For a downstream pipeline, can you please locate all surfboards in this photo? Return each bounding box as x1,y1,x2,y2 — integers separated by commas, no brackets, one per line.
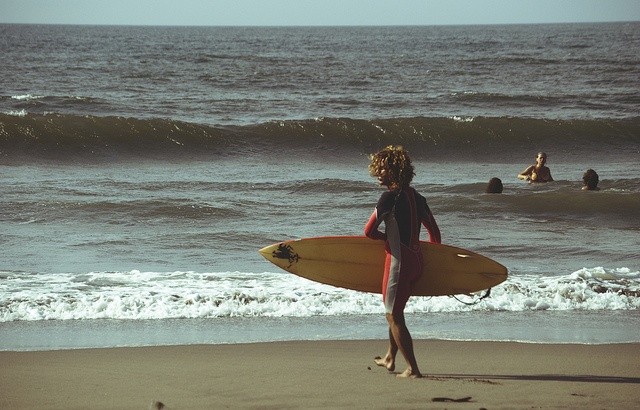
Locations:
258,235,509,297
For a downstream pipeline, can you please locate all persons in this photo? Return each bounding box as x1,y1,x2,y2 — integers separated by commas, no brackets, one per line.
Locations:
518,153,555,184
365,145,442,379
581,168,602,191
485,176,503,193
